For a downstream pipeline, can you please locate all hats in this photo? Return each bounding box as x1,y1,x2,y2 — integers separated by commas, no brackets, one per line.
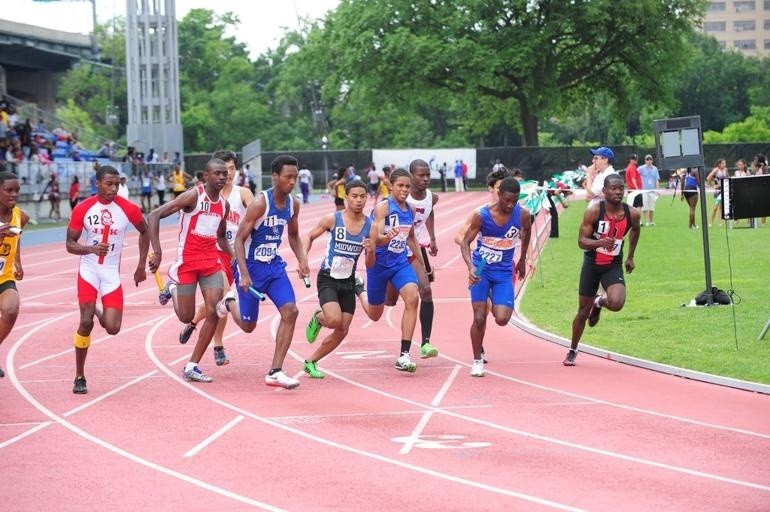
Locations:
589,147,615,162
628,153,638,160
644,155,653,160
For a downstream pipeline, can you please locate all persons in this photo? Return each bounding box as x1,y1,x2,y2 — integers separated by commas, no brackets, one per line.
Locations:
237,164,257,197
295,162,398,204
561,173,642,367
196,170,207,185
667,176,681,190
146,157,236,384
459,174,534,378
439,160,470,192
453,166,538,368
354,166,428,373
301,178,379,379
215,153,311,389
66,163,151,395
0,168,31,378
177,148,257,368
680,153,769,229
492,147,617,209
402,158,439,360
2,91,194,222
626,153,660,227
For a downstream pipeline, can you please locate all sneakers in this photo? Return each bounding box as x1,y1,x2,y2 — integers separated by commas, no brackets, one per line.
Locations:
73,376,88,394
395,343,439,373
303,307,327,380
481,352,488,363
158,274,239,384
563,350,578,366
587,295,602,328
470,359,484,377
264,369,300,390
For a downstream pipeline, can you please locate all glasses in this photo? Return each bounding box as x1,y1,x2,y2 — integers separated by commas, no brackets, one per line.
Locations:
647,158,653,160
